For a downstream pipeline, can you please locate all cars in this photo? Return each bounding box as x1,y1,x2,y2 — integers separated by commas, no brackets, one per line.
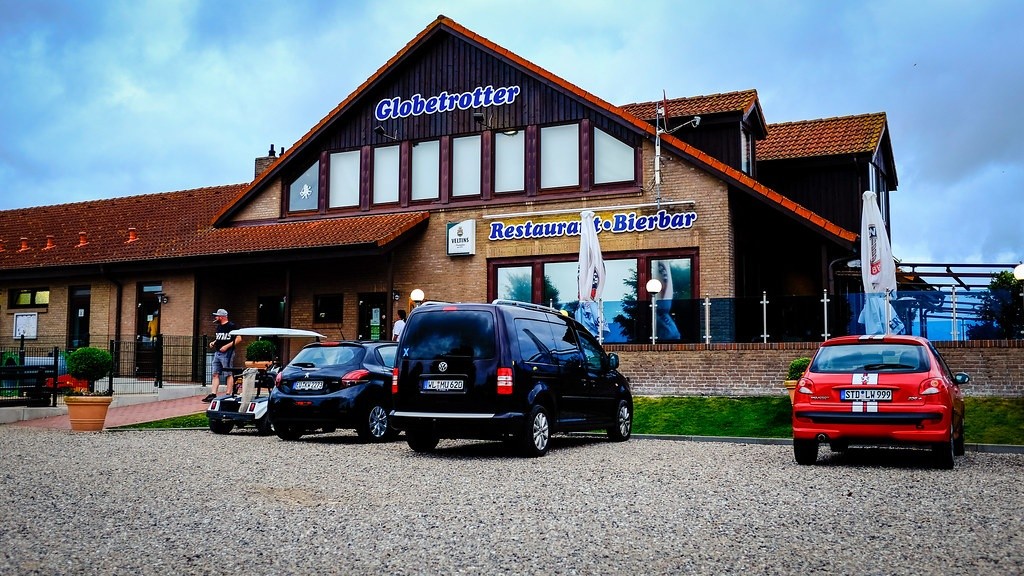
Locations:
266,340,400,443
793,335,970,469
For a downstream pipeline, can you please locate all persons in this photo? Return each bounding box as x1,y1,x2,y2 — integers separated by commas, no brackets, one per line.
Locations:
202,309,242,402
392,310,406,342
148,309,158,342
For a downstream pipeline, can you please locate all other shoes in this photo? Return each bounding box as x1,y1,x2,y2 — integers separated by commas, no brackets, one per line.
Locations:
202,394,216,403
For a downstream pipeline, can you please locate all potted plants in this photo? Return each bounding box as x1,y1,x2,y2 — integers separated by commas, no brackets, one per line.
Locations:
244,340,277,370
784,357,813,407
64,348,113,432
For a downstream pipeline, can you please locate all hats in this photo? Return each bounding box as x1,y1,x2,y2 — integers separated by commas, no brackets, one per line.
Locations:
212,309,229,316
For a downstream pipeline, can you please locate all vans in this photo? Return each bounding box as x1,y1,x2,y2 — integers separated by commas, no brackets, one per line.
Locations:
388,298,634,456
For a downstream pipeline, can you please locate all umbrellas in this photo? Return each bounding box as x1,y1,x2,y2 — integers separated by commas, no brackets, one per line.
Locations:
575,211,610,335
858,191,904,335
651,260,679,339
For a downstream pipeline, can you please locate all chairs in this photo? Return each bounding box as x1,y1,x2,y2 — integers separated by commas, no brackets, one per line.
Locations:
900,350,919,369
862,353,886,367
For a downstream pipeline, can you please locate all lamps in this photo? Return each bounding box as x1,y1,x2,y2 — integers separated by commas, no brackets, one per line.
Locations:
646,279,662,297
410,289,425,305
154,292,168,304
374,125,398,141
1014,264,1024,286
474,113,492,129
